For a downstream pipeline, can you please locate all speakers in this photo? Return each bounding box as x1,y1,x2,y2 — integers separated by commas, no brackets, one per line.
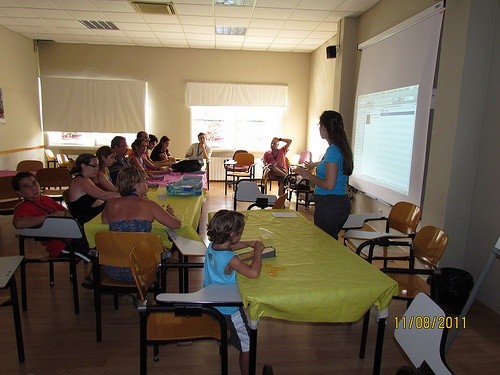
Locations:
326,45,336,58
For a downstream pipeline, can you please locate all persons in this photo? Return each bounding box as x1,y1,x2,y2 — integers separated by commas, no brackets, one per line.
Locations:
203,209,264,375
297,110,353,242
128,131,176,180
100,167,180,307
12,172,93,288
69,153,120,225
184,133,212,160
263,137,296,197
96,136,131,187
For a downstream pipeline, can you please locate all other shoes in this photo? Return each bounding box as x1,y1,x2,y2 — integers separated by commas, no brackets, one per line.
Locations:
81,275,101,289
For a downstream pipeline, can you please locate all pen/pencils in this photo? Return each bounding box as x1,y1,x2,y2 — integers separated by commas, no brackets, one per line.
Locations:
162,204,168,208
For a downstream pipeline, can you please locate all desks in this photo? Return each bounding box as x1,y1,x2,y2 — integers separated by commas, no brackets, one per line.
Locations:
208,208,398,375
84,169,206,299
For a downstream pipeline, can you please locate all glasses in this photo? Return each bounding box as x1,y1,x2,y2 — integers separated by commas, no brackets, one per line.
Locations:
271,142,277,145
144,137,151,141
152,142,155,146
200,137,205,140
120,145,128,148
87,163,101,168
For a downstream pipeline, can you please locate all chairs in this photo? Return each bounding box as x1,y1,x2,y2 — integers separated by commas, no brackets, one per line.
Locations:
339,202,422,314
224,152,255,195
16,215,91,315
359,225,449,375
89,232,168,344
283,151,317,211
129,241,245,375
0,160,72,216
0,255,28,363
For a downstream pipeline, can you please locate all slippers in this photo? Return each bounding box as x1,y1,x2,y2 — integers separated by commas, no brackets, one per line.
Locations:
288,177,296,184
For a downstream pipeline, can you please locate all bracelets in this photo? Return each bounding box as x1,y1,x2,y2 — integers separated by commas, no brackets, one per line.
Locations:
280,138,281,140
